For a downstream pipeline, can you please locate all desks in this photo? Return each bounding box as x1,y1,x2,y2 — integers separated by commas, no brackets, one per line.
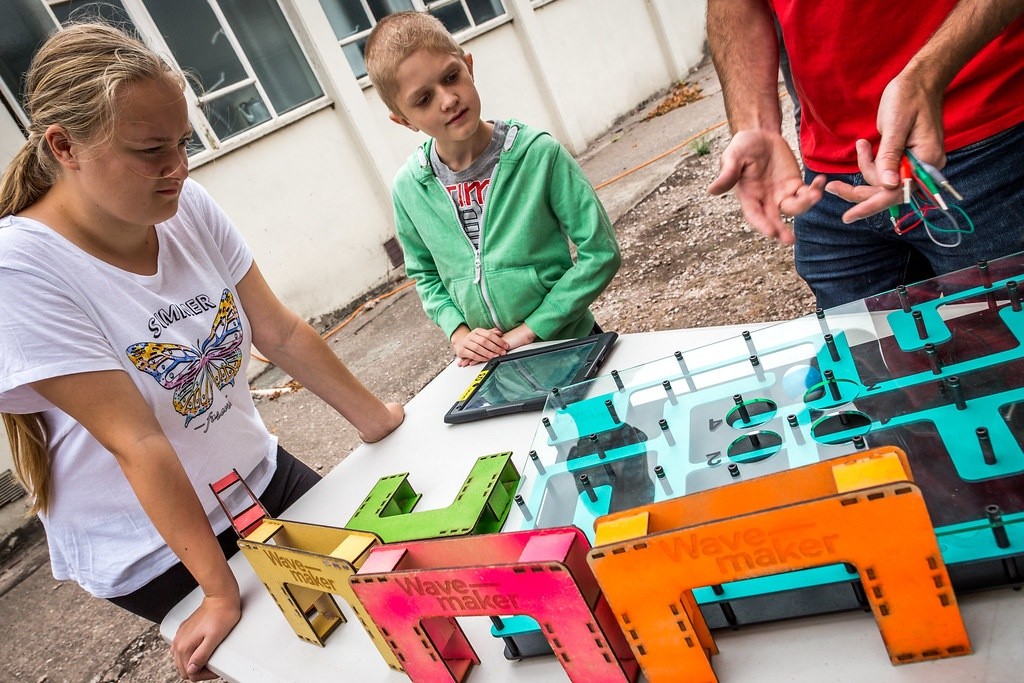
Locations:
156,299,1024,683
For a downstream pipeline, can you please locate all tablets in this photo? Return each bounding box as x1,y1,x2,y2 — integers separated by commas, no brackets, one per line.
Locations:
444,330,619,424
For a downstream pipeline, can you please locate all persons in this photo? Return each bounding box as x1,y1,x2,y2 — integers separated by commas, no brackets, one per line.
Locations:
1,23,404,682
707,0,1024,311
365,11,622,367
